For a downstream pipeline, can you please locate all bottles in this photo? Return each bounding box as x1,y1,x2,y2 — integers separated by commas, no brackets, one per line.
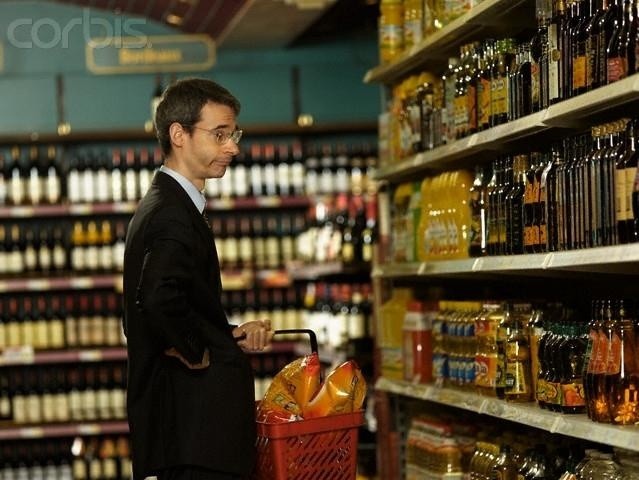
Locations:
0,133,377,480
372,0,639,480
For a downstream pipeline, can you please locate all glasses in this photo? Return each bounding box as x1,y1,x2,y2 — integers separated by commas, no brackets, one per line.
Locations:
181,123,243,145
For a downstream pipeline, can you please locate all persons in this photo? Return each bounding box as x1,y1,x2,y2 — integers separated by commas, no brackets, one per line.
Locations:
120,77,277,480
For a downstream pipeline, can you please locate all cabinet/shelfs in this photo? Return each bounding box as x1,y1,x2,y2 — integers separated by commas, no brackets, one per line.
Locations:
0,116,378,479
362,0,637,478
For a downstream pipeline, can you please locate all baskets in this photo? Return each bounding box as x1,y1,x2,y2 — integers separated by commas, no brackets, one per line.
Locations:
235,328,365,480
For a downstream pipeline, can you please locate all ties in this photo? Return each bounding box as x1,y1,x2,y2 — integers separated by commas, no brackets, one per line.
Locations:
201,206,211,230
